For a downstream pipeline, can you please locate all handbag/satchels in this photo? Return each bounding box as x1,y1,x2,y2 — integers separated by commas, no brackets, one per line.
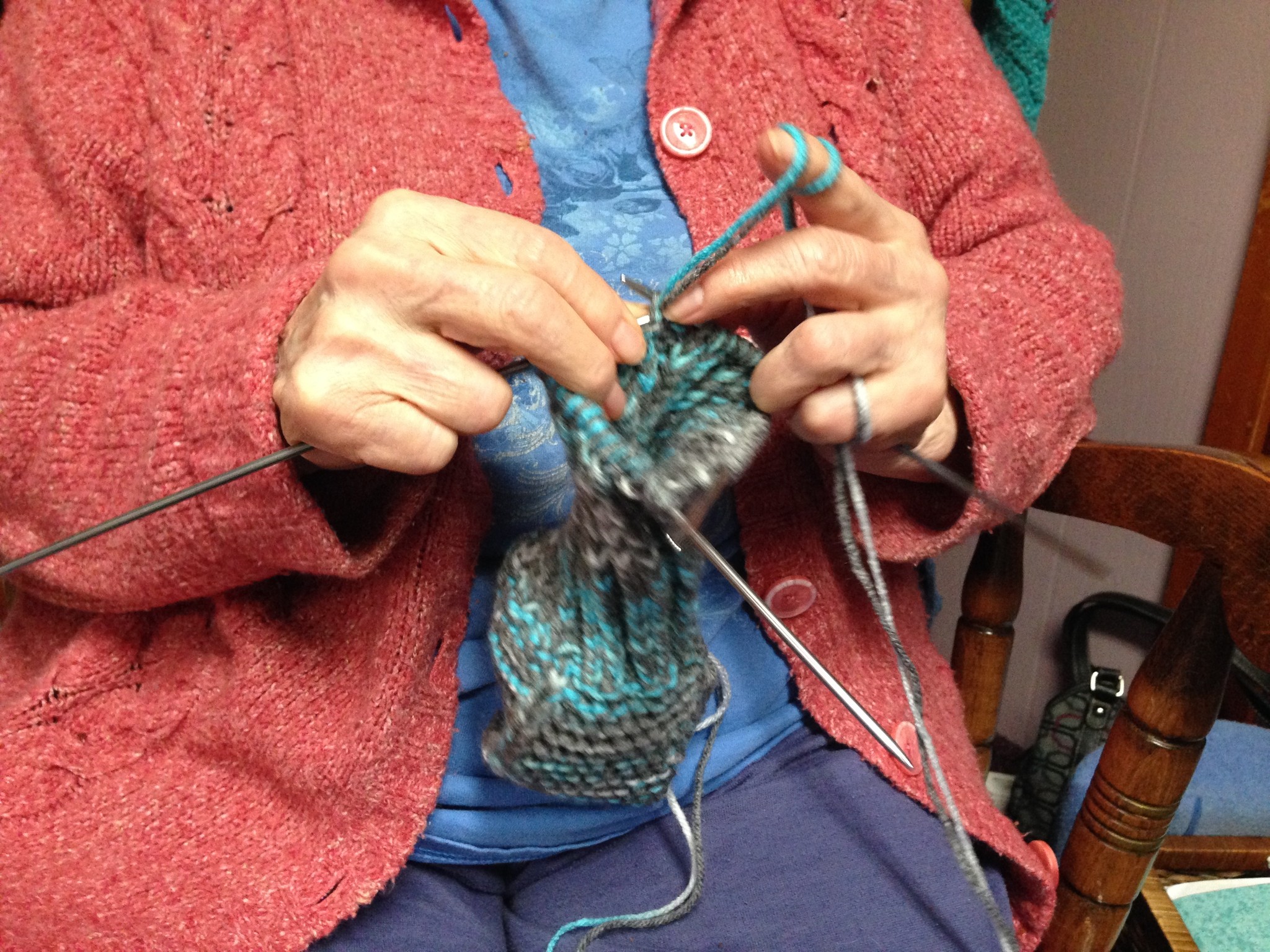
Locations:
1005,590,1270,846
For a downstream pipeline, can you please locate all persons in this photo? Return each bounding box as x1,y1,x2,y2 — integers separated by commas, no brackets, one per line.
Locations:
0,0,1127,952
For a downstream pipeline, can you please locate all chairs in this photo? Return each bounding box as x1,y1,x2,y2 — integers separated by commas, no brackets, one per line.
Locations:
947,431,1270,952
